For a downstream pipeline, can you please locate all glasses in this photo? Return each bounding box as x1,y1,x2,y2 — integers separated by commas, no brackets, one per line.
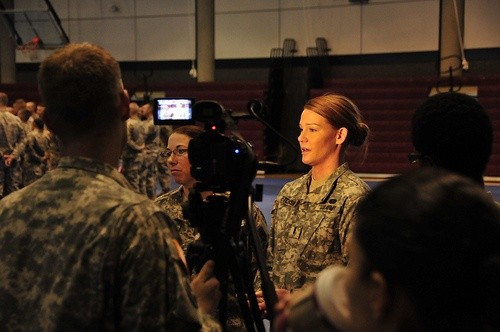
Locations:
407,153,422,167
162,147,190,156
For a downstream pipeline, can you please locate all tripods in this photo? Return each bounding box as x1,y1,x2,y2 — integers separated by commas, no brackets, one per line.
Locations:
181,181,279,332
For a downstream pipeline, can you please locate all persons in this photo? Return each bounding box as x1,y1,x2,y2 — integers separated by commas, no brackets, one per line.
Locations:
253,92,375,332
151,127,269,332
276,92,500,332
0,43,222,332
0,93,174,200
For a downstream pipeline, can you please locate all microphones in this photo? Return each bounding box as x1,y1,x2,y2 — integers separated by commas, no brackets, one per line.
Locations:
248,99,269,117
